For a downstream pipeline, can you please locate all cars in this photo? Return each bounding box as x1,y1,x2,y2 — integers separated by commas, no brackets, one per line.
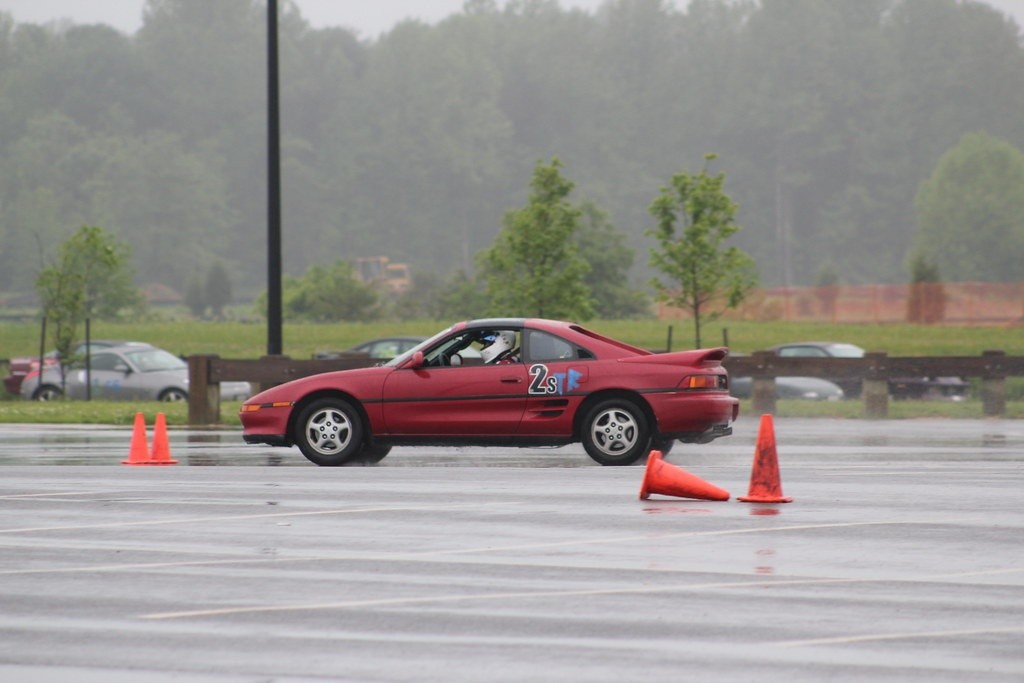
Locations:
239,318,740,467
316,336,482,358
4,340,250,401
730,342,968,403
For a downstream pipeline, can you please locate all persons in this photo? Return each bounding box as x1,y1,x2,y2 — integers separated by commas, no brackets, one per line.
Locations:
475,329,519,365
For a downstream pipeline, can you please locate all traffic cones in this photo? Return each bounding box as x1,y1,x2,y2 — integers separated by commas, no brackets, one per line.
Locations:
735,415,791,503
150,412,178,464
121,412,150,465
640,450,730,502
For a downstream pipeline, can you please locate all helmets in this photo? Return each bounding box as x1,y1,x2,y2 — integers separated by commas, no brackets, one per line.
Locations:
479,327,517,364
